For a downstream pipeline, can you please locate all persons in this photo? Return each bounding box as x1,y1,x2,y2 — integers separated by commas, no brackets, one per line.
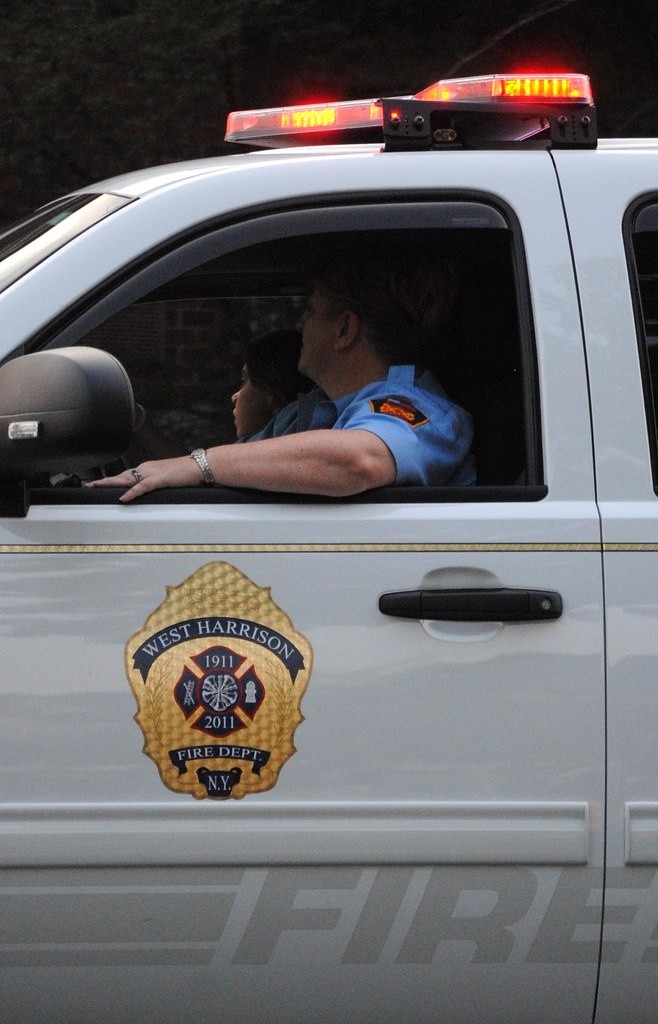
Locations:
83,266,476,502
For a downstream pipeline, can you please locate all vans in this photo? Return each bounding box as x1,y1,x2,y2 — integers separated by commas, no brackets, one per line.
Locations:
0,72,658,1024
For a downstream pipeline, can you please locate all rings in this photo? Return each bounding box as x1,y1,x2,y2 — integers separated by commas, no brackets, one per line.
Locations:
131,468,139,481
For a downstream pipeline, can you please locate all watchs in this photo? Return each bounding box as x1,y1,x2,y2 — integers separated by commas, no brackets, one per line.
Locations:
191,447,215,483
